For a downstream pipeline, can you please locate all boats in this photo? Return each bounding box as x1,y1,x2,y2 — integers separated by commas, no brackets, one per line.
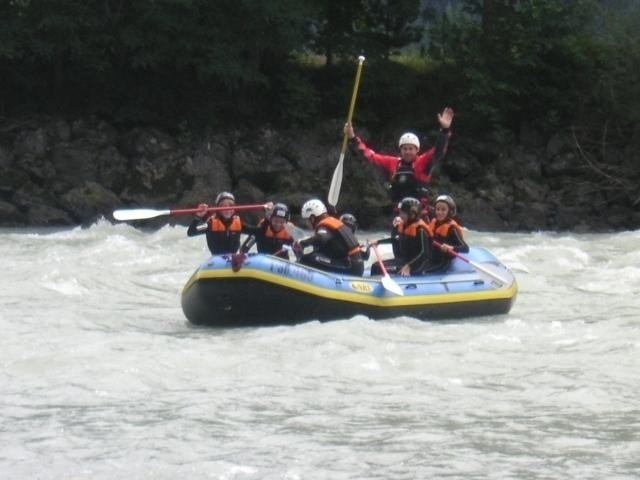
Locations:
179,238,521,333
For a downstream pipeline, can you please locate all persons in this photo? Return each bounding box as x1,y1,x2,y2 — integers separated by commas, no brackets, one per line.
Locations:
186,191,276,255
338,212,370,262
344,108,455,257
240,201,305,264
291,197,366,278
428,193,469,274
368,196,436,277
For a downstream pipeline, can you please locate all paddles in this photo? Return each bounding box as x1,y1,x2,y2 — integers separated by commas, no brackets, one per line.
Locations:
327,56,368,206
373,244,404,296
111,202,273,222
433,241,513,290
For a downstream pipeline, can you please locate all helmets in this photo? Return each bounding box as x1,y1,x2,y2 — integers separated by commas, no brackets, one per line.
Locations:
215,192,235,206
433,195,456,218
271,203,290,224
339,214,357,233
301,199,328,219
399,132,420,150
398,197,422,223
